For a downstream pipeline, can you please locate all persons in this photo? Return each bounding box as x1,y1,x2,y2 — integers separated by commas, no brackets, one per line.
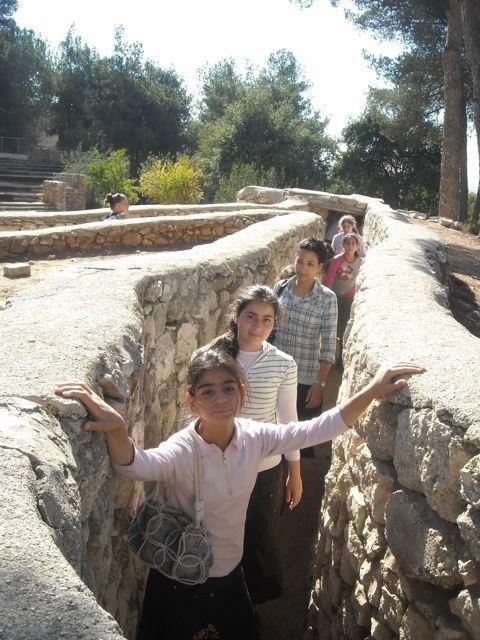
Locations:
55,351,426,640
330,214,363,256
191,286,303,605
104,193,129,219
326,234,364,372
271,238,338,458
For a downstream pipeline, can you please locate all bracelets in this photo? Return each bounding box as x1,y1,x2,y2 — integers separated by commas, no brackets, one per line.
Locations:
314,379,328,388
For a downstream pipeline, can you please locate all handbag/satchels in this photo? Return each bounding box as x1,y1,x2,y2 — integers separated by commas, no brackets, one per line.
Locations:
126,492,213,586
